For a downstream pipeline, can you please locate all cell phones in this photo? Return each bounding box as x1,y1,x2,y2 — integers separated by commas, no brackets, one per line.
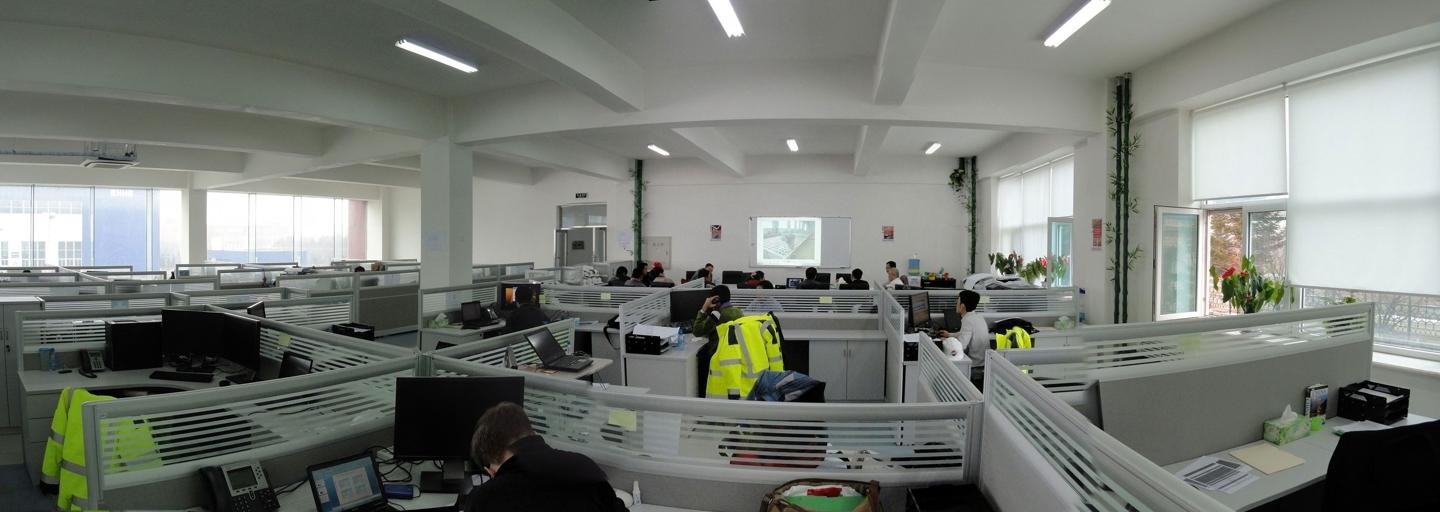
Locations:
713,298,719,304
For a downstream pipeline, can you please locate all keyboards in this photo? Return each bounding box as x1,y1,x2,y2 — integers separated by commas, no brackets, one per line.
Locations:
150,371,214,383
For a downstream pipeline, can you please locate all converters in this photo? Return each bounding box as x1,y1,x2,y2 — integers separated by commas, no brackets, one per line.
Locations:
385,483,414,499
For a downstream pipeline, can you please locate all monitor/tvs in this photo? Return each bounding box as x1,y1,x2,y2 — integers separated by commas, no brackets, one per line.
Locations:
392,375,527,494
247,299,267,318
162,306,222,372
908,293,931,330
670,289,712,322
499,281,541,317
686,271,852,288
217,322,262,383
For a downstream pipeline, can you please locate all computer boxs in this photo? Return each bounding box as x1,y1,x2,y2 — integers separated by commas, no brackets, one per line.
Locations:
106,319,162,372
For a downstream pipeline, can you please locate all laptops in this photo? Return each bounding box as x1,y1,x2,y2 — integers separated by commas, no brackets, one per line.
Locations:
306,451,402,512
461,300,499,327
278,350,313,379
524,327,593,370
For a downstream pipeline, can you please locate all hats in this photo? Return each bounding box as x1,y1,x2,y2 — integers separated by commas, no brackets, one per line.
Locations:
942,337,964,361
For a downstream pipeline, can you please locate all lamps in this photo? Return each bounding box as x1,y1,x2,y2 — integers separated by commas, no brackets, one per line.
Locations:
1041,0,1112,48
394,38,482,76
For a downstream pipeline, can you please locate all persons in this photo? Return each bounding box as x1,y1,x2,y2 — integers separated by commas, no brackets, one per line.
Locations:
745,280,785,312
743,270,765,286
465,400,629,511
882,261,904,289
846,267,869,290
503,285,546,332
608,261,674,287
698,268,715,288
796,267,828,289
355,266,365,272
692,283,744,356
941,290,994,383
689,263,714,281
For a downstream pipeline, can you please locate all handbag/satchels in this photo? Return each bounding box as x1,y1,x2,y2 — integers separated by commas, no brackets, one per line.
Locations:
761,477,880,512
719,370,826,467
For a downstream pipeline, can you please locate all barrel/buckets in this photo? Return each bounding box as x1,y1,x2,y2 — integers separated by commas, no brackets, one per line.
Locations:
908,258,921,276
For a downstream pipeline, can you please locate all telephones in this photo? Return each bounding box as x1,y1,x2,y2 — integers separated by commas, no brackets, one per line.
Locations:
697,297,720,308
80,349,105,372
199,458,281,512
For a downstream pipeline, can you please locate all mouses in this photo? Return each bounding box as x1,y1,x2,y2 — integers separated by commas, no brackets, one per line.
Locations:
220,380,230,386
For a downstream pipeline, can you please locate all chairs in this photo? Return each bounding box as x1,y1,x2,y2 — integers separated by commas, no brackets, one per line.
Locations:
1,256,1438,512
